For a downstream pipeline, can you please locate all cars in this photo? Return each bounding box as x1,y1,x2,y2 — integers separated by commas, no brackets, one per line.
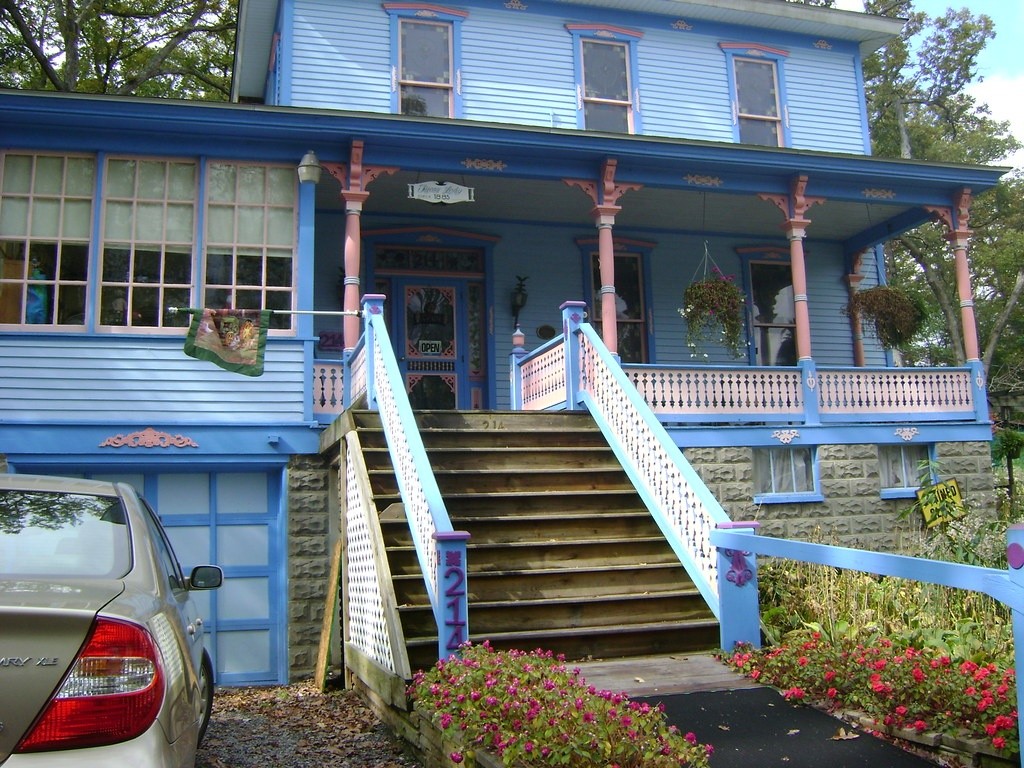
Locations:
0,473,225,768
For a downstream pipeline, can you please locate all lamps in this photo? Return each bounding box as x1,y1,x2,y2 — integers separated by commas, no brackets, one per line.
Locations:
296,149,324,184
338,267,345,310
510,275,530,328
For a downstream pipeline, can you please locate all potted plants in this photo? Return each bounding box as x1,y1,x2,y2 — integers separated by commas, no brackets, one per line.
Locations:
839,288,930,351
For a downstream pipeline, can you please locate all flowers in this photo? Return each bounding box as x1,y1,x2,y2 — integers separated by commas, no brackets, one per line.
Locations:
676,267,751,364
